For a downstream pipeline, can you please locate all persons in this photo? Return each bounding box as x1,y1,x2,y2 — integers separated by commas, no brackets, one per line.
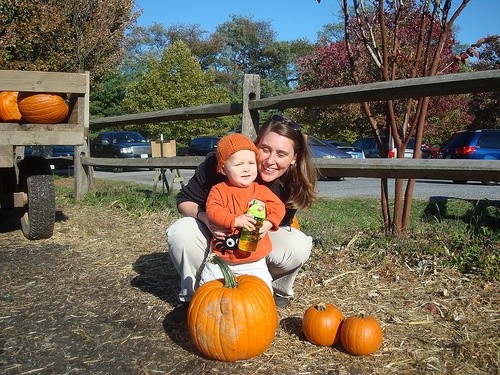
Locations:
198,132,286,296
165,113,318,323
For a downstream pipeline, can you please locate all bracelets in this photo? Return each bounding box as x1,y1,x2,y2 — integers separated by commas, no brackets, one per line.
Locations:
196,210,200,220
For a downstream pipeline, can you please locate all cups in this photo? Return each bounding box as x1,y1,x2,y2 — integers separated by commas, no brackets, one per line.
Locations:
237,199,266,252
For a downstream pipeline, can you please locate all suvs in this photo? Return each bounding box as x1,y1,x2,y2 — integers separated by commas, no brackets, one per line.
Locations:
438,129,500,183
357,135,417,160
189,136,220,156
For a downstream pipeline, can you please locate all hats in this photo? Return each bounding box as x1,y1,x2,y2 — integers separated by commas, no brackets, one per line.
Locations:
216,132,259,172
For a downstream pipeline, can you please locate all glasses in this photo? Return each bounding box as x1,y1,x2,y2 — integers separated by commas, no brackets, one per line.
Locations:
271,114,300,131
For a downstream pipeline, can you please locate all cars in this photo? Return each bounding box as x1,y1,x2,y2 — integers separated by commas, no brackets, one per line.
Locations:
325,140,364,158
306,134,349,180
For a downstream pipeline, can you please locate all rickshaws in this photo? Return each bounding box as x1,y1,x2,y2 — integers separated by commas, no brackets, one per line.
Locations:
0,69,90,239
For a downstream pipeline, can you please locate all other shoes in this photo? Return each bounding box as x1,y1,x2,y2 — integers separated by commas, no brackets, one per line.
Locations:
274,291,289,307
173,301,187,324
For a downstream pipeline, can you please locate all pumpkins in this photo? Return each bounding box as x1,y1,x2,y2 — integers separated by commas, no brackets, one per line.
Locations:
17,91,69,124
187,254,277,362
302,302,344,347
0,91,26,123
341,313,382,355
291,216,299,230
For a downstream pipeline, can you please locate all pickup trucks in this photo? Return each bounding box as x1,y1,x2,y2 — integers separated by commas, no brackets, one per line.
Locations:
87,131,156,172
